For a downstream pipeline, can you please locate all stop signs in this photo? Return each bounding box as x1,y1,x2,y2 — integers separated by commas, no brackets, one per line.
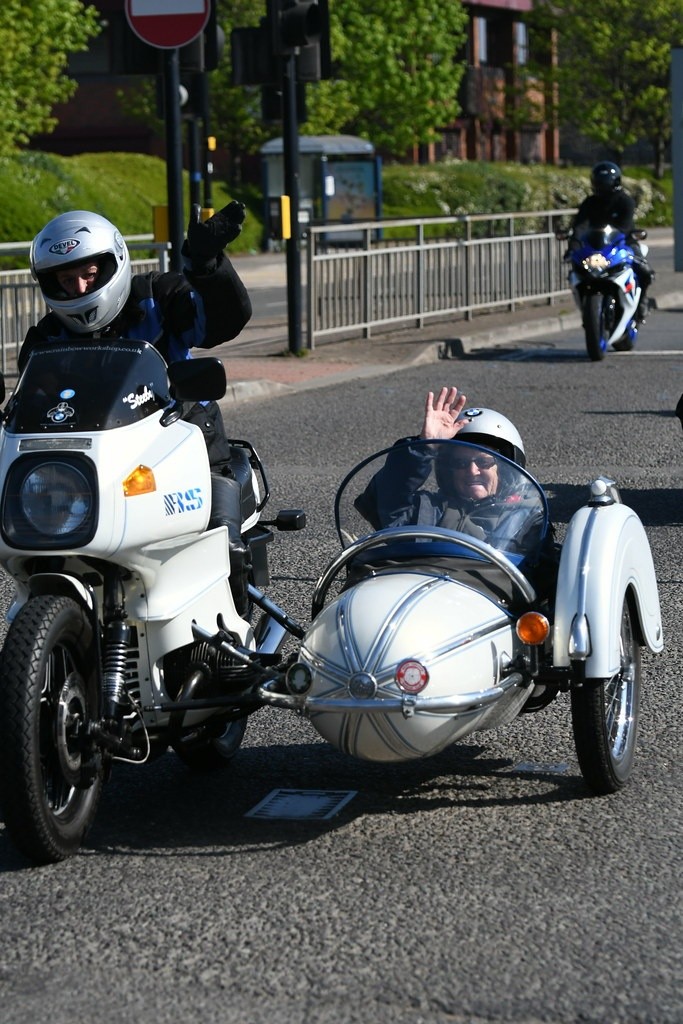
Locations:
125,0,211,50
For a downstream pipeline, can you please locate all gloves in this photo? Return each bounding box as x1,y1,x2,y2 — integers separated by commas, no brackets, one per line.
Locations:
181,201,245,276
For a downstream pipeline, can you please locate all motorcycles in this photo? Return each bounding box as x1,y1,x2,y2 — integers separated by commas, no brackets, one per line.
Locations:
554,227,659,361
1,337,665,864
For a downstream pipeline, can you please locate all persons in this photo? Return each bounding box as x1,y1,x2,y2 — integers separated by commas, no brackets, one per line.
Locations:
562,161,655,319
19,201,253,619
353,385,555,560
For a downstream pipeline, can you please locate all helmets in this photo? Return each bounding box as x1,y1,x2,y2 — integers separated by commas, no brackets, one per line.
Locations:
29,212,132,334
434,407,526,501
591,161,621,194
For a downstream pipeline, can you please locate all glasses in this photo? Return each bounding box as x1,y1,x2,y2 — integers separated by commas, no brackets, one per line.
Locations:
447,454,498,469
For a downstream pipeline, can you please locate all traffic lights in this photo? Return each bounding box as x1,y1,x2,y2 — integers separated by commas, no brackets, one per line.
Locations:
279,0,324,46
